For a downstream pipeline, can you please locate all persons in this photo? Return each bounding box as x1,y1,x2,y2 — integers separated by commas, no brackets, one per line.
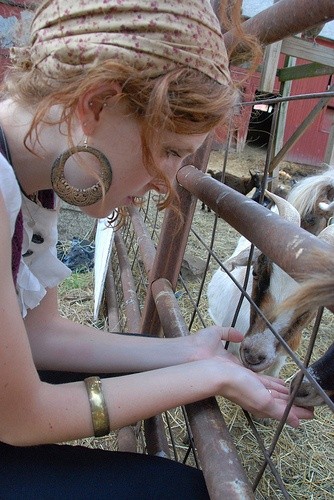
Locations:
0,0,315,499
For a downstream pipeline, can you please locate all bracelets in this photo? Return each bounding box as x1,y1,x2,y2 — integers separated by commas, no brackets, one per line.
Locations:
84,375,111,439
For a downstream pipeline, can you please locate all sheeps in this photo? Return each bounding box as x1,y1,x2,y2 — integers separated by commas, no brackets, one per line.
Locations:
289,342,334,407
205,169,334,426
201,170,265,218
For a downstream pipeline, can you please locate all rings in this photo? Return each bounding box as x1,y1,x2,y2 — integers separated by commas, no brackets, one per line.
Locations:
267,389,272,395
